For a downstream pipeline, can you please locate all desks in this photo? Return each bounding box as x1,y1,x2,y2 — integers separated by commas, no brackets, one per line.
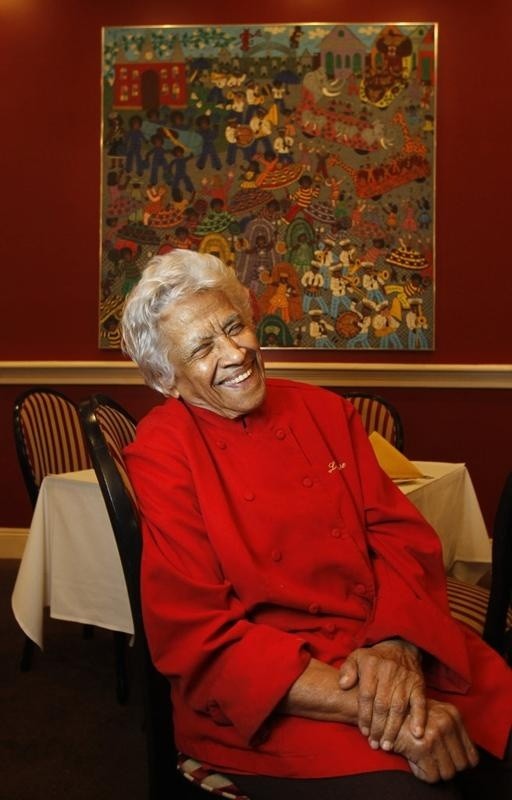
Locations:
42,460,466,707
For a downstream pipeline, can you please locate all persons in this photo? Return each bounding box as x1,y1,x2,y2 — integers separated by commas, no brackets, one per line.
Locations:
120,246,512,799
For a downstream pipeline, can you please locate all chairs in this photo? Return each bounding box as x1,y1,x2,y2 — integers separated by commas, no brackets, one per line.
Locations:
82,400,150,575
14,394,112,646
424,481,512,698
344,396,402,452
